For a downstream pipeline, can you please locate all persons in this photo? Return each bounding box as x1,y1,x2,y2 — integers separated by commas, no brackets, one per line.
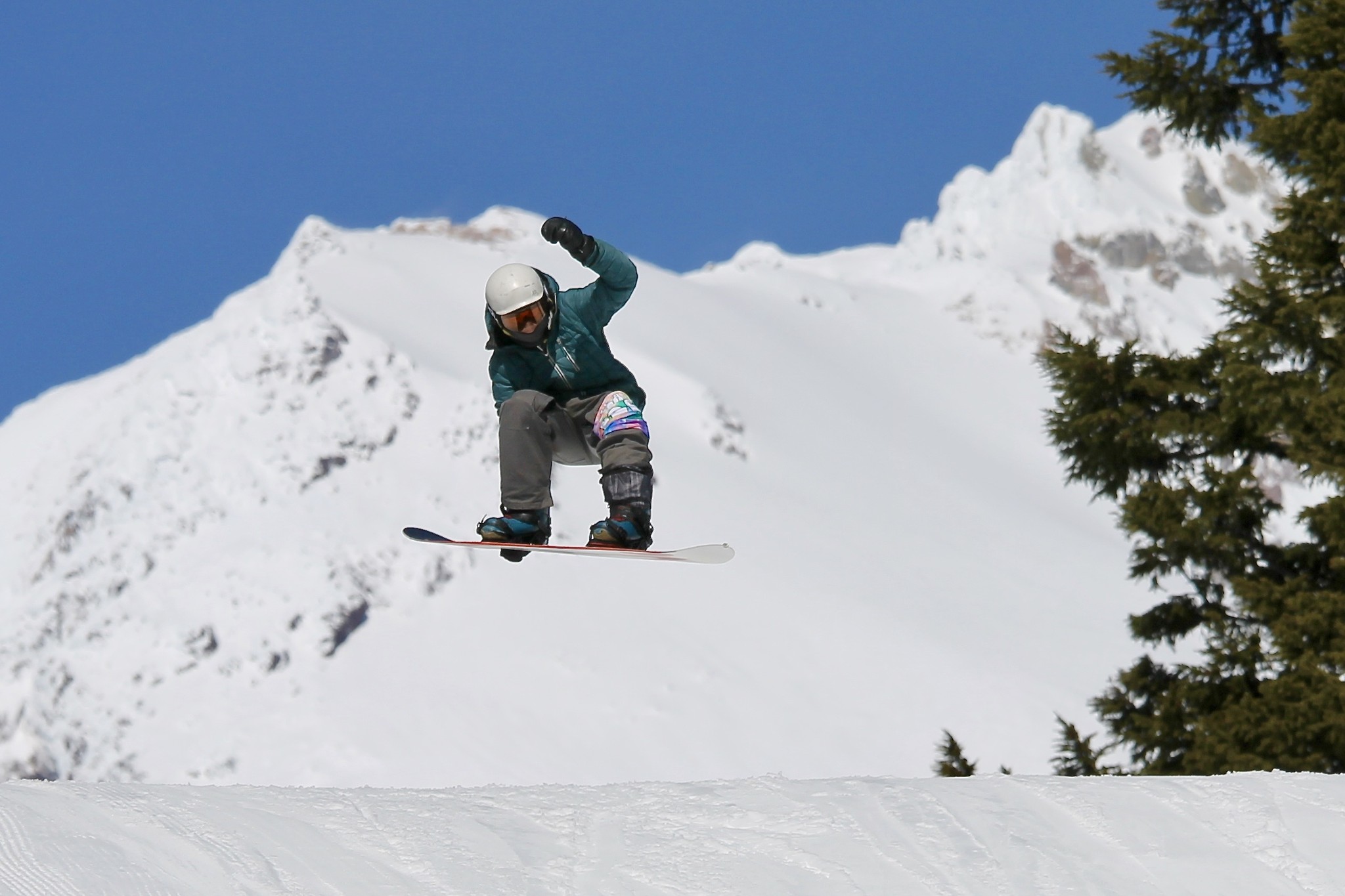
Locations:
476,216,654,550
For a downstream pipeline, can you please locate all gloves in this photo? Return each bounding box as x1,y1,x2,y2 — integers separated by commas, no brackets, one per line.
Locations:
542,217,594,261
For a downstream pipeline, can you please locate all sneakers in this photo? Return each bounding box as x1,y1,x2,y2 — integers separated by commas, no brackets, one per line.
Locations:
480,509,549,544
591,505,653,543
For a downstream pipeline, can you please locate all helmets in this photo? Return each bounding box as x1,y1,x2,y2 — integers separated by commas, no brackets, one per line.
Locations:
486,263,543,315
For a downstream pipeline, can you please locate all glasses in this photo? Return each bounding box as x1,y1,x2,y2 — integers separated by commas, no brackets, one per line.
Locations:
496,298,547,331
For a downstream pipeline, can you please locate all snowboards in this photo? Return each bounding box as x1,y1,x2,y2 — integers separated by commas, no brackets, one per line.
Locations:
401,525,738,565
